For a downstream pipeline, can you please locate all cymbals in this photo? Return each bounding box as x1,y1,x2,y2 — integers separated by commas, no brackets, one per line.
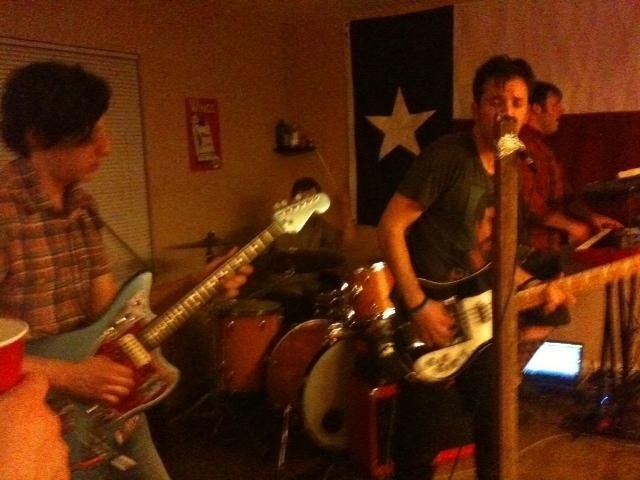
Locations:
264,245,344,275
162,239,246,248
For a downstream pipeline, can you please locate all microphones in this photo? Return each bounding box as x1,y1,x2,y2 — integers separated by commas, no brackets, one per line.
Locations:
518,140,540,173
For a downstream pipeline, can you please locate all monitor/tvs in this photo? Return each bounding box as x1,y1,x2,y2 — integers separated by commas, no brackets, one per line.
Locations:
522,339,585,393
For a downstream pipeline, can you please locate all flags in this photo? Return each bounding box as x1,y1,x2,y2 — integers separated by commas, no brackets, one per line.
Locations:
345,0,456,227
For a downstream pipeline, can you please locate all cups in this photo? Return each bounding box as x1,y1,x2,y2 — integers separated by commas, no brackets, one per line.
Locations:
0,318,30,395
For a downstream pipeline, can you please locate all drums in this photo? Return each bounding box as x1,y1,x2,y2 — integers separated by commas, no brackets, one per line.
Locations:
313,262,400,344
263,319,373,451
200,299,283,393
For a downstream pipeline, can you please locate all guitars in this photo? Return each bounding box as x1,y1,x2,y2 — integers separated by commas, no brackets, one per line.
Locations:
24,188,331,479
388,253,639,382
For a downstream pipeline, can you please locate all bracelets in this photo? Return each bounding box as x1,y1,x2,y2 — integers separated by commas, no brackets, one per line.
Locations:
404,295,430,316
515,277,540,291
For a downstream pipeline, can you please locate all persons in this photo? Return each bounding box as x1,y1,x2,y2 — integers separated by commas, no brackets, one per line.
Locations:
374,51,578,479
513,78,628,400
269,175,352,327
0,58,256,478
